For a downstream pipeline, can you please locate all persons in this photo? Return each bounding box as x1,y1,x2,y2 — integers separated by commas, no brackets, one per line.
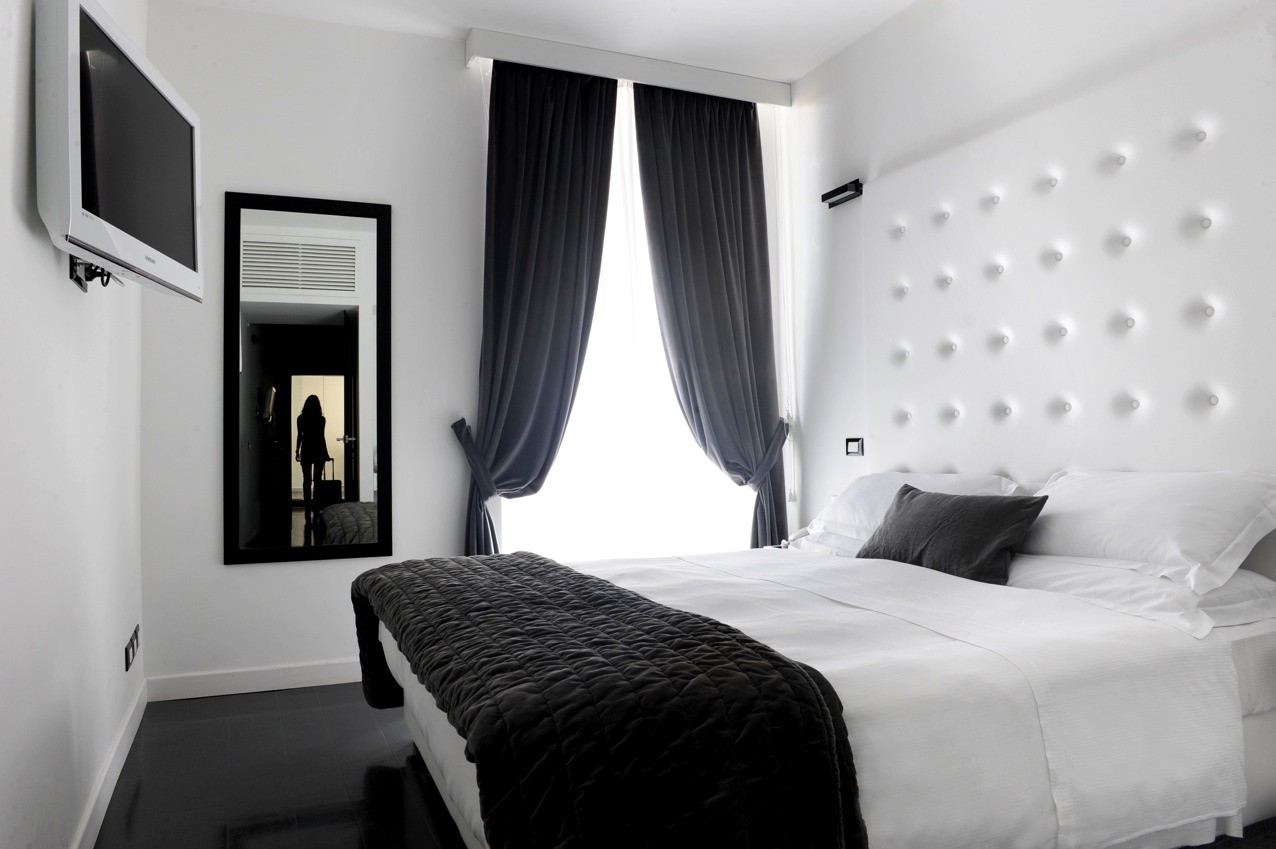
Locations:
295,395,333,523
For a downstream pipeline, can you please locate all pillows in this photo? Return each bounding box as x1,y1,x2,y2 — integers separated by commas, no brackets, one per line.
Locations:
791,472,1276,638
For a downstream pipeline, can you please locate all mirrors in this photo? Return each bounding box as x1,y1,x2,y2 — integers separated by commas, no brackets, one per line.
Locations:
224,191,393,563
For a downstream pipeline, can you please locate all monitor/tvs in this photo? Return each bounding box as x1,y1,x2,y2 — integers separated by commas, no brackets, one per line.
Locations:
32,0,204,304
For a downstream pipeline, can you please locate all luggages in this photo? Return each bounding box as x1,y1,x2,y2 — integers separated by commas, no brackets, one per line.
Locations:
320,458,342,505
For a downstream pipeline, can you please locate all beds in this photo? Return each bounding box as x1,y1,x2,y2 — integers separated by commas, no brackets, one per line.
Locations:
352,468,1276,849
315,501,378,546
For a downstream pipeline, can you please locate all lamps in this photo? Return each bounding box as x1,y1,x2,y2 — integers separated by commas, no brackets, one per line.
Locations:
821,178,863,209
256,384,276,423
